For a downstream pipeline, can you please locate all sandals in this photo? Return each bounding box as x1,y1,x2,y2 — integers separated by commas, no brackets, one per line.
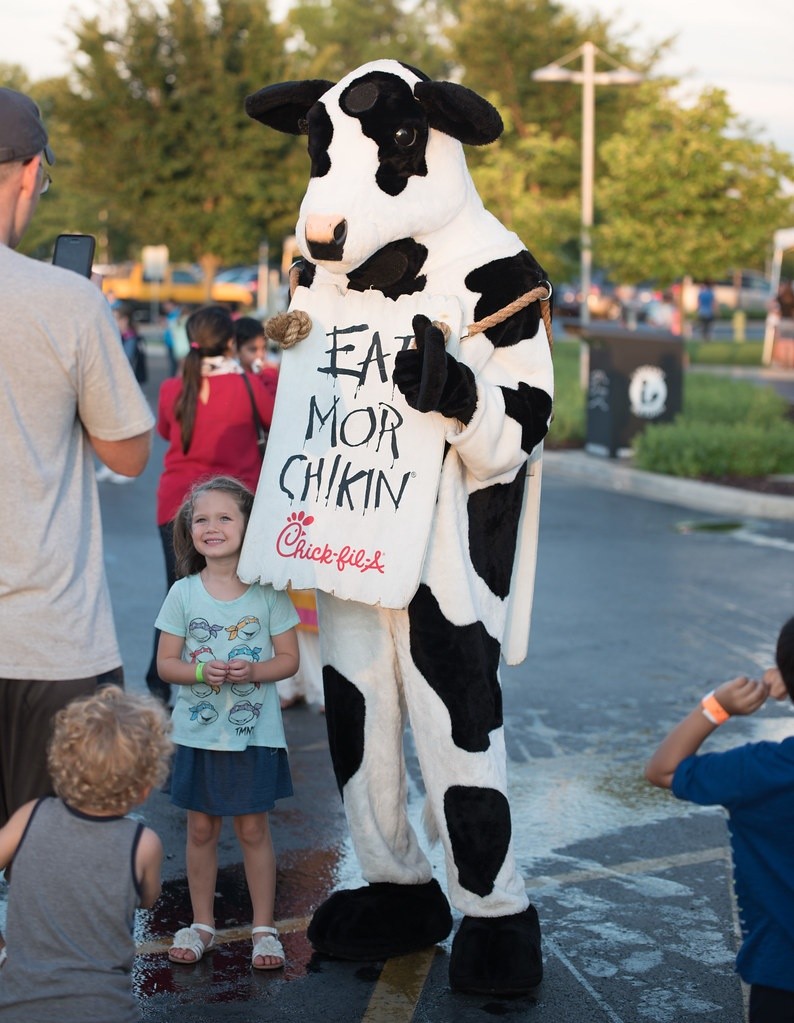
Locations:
252,926,285,969
168,924,215,962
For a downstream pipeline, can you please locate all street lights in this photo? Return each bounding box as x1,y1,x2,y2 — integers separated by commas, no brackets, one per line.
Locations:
532,44,642,388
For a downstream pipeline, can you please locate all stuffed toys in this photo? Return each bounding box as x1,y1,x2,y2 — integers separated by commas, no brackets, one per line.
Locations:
269,58,555,1002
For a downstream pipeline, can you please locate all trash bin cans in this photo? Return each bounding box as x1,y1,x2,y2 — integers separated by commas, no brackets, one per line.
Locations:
583,333,687,459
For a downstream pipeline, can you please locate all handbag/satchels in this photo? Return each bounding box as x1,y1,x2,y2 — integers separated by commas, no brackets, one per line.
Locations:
257,429,268,462
135,338,147,383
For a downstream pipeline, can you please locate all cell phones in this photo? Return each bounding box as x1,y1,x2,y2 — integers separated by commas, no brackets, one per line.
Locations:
51,234,95,278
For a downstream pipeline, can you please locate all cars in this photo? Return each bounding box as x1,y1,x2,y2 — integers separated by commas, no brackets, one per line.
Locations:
698,273,773,319
209,266,260,300
102,261,253,317
559,268,657,321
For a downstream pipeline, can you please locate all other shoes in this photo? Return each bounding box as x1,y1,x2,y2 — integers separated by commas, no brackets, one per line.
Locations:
96,465,135,485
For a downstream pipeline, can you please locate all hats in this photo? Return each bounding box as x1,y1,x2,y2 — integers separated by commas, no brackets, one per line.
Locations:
0,86,55,166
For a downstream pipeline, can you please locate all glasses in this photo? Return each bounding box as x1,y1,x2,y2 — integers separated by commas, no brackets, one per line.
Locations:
22,157,52,196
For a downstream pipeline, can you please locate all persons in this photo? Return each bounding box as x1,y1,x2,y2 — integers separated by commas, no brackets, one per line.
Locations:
1,86,301,1023
697,278,714,344
645,617,794,1023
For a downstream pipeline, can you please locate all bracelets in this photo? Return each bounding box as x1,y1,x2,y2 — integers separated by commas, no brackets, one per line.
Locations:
195,662,205,682
700,690,728,727
